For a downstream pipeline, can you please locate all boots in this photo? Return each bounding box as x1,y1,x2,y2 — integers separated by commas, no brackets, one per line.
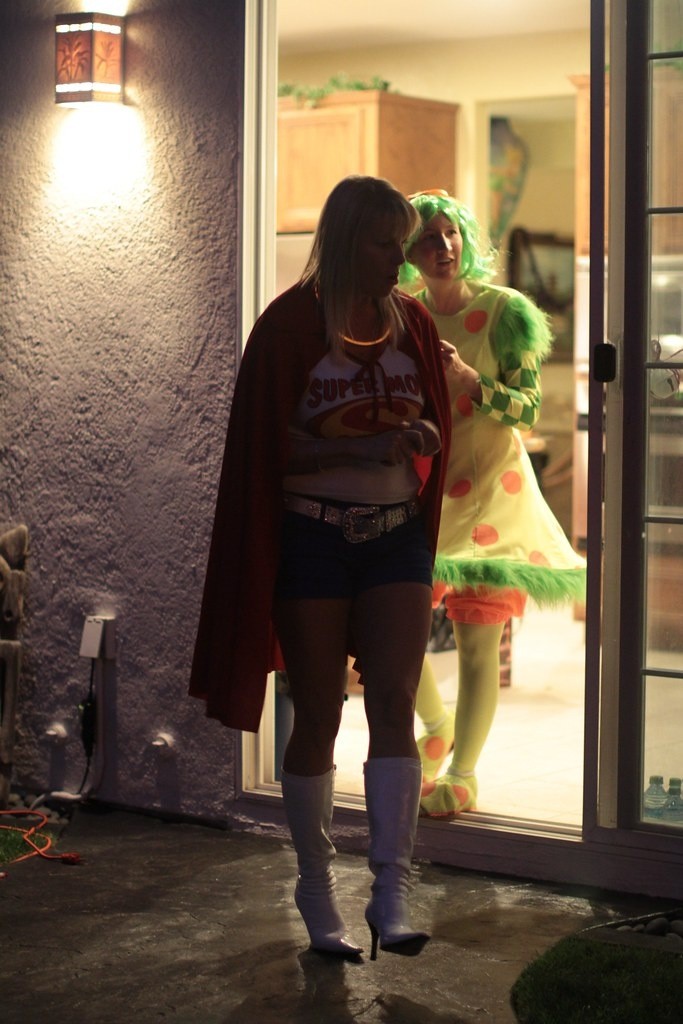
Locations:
280,768,363,957
362,757,430,961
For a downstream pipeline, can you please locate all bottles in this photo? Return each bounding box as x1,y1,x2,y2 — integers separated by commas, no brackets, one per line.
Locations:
662,786,683,822
642,775,666,819
666,777,683,800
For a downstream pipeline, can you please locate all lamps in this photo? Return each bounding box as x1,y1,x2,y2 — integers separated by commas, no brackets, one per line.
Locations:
53,13,127,107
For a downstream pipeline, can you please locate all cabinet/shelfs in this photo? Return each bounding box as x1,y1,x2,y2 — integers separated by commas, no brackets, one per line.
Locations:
275,90,460,234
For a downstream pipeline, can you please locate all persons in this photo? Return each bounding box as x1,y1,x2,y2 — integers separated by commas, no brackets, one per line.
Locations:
391,185,586,819
185,176,455,960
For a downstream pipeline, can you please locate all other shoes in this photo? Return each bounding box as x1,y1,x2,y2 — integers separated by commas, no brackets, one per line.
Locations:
419,774,477,816
416,717,454,783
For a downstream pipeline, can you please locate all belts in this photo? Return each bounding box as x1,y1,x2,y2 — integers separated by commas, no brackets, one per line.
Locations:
283,494,423,542
